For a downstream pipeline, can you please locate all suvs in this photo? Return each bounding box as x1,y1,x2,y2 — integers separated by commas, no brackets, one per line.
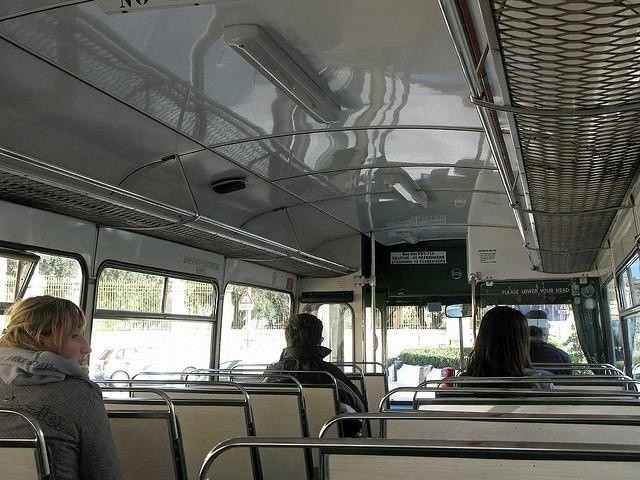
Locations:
93,344,205,390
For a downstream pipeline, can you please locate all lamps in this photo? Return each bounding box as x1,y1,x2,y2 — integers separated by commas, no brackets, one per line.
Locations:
223,24,344,125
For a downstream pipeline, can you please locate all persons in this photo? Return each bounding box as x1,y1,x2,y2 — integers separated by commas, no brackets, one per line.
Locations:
0,292,124,479
450,305,555,398
523,308,573,377
253,311,368,437
